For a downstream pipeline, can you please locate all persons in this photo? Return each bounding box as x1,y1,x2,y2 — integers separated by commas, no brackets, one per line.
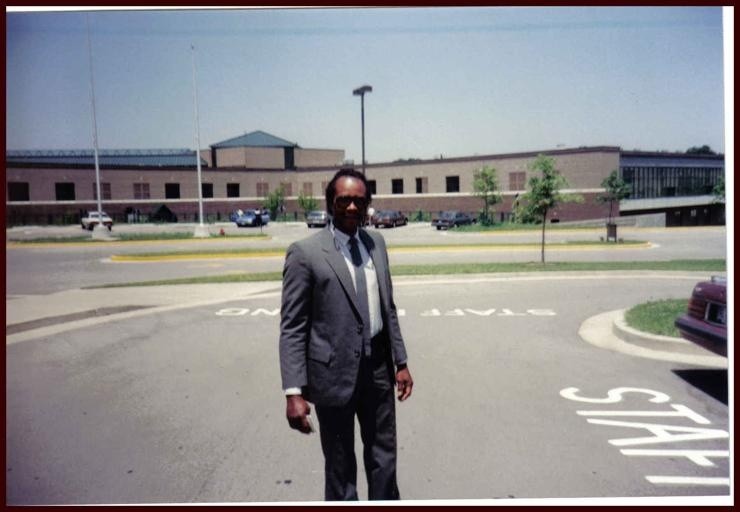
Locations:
278,169,412,500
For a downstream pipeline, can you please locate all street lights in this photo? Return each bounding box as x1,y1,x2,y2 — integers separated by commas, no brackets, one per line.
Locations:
353,85,372,177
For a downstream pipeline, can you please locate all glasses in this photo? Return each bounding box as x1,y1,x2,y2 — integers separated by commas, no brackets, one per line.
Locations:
333,195,371,210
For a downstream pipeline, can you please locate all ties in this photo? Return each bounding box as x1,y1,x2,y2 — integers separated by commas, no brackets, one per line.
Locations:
346,237,372,360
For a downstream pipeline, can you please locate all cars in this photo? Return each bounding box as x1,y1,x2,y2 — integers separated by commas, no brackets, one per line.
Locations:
307,207,476,229
80,212,113,230
674,275,727,361
228,205,287,229
